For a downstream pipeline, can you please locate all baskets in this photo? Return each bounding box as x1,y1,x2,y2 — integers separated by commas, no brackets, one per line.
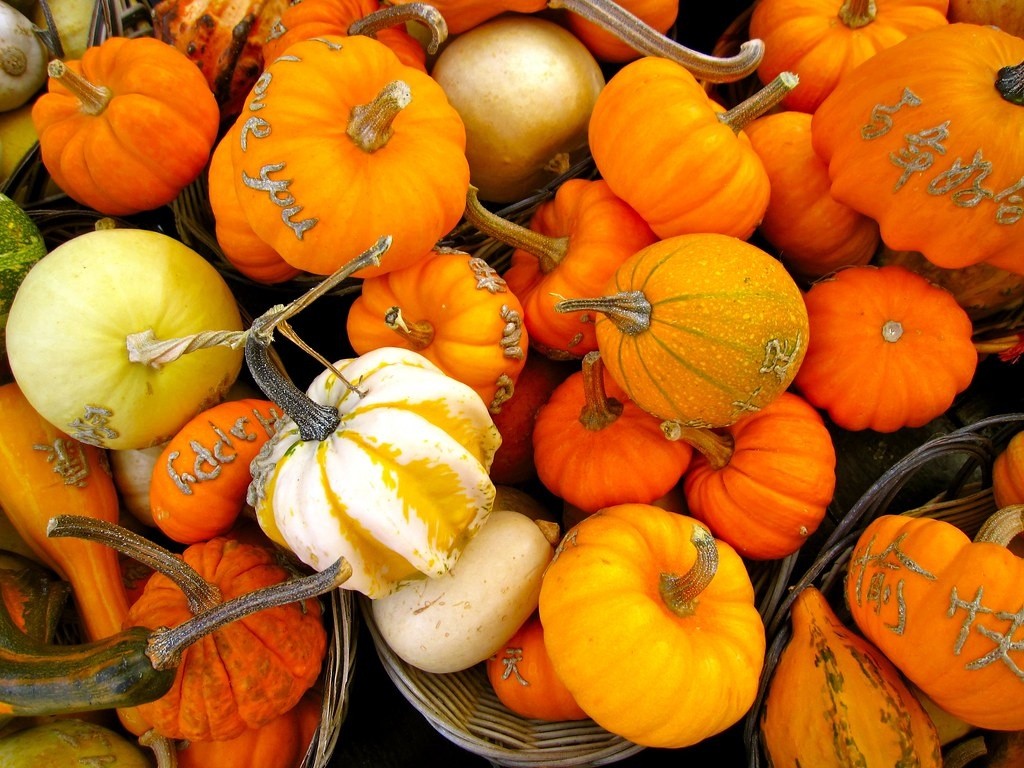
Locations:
1,0,117,202
699,0,1023,357
740,410,1023,768
92,0,676,291
358,198,802,768
1,208,357,768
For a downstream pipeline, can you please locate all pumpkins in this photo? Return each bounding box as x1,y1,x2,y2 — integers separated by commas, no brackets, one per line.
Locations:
1,1,1024,768
